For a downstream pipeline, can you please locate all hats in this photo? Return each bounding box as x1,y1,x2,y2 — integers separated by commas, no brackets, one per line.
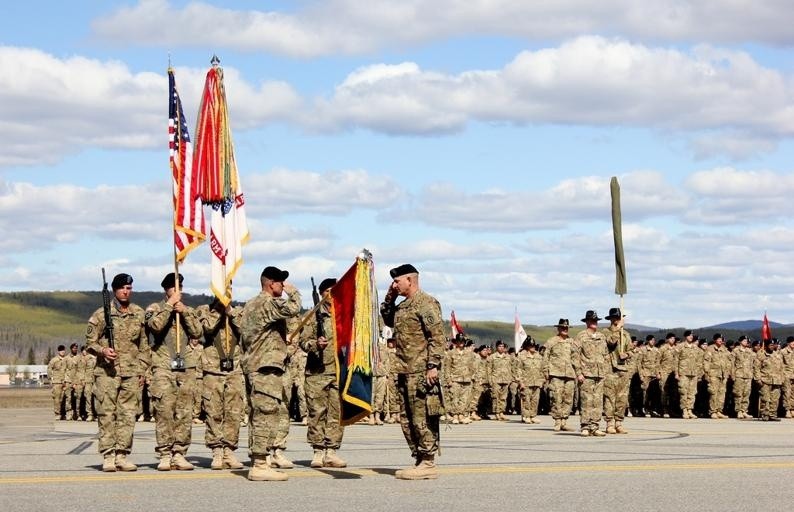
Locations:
58,346,65,351
70,343,78,349
161,273,183,288
262,266,289,282
522,335,546,352
554,319,573,328
605,308,626,320
112,274,133,288
319,278,336,294
446,333,515,354
581,311,602,322
708,333,794,346
631,329,707,347
390,264,418,279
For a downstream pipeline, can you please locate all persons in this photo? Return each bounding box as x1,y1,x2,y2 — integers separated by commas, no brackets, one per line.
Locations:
49,342,99,421
137,377,156,422
361,335,401,424
380,263,447,479
298,278,347,469
239,375,251,426
541,317,574,431
190,337,205,424
602,308,633,433
238,266,304,481
285,353,308,423
571,310,610,436
625,329,793,423
144,272,206,471
439,334,554,424
84,273,151,472
196,296,245,470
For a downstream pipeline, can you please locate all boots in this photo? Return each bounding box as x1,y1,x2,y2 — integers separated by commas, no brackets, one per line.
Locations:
369,412,400,426
158,452,173,471
395,455,437,479
581,427,589,436
682,409,794,421
590,428,606,436
193,418,204,425
310,450,323,468
171,453,193,470
211,447,223,470
56,413,95,422
521,416,540,424
440,412,482,424
103,454,116,472
325,449,347,468
553,418,570,431
615,422,628,434
496,412,509,421
115,453,137,471
248,449,293,481
223,446,244,470
607,421,616,434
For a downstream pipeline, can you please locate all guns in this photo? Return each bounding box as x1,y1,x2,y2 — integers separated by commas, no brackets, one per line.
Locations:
100,268,117,377
308,276,326,373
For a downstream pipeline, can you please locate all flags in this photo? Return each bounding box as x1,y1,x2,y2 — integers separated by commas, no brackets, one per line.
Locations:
330,259,372,424
168,69,205,263
450,310,463,337
191,67,249,307
761,311,772,340
